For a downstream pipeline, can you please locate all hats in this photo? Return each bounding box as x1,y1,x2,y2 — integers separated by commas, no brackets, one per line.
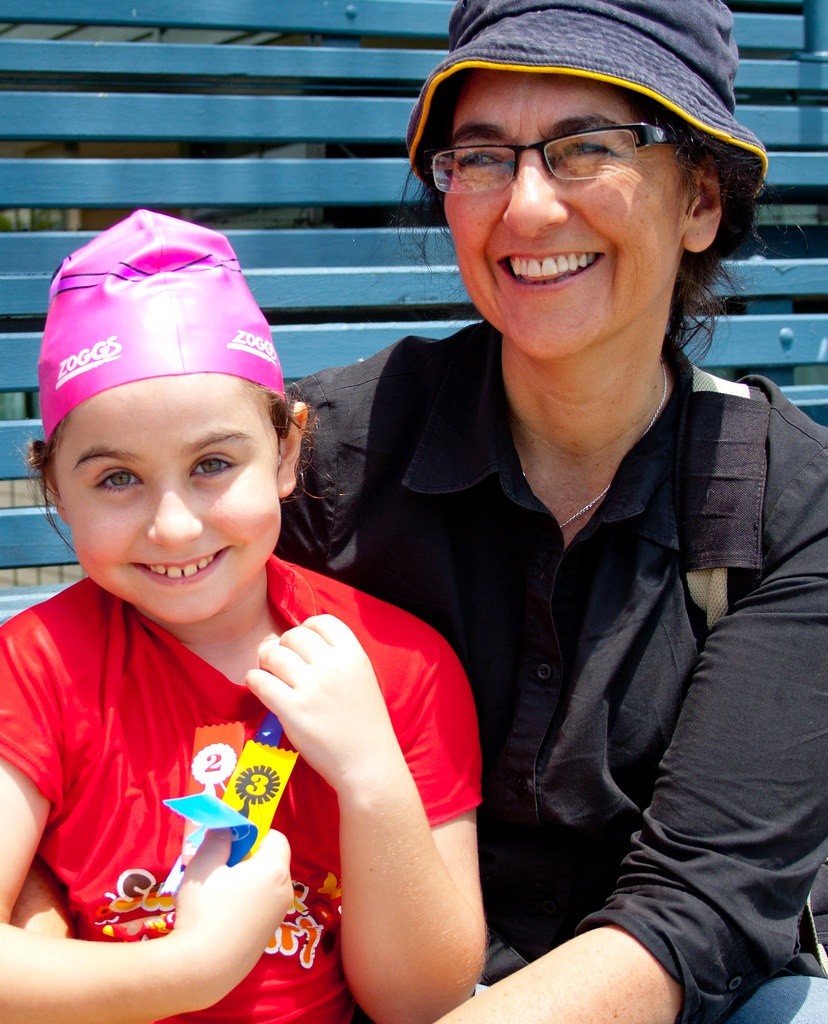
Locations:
407,0,769,197
39,210,284,442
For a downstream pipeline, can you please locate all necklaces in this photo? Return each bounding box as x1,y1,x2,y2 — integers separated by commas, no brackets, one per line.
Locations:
558,349,671,530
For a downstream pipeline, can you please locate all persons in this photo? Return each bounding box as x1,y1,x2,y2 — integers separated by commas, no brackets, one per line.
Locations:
0,198,489,1024
277,0,827,1024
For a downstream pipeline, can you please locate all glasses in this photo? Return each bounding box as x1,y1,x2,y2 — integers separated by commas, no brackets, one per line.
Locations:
424,122,689,194
